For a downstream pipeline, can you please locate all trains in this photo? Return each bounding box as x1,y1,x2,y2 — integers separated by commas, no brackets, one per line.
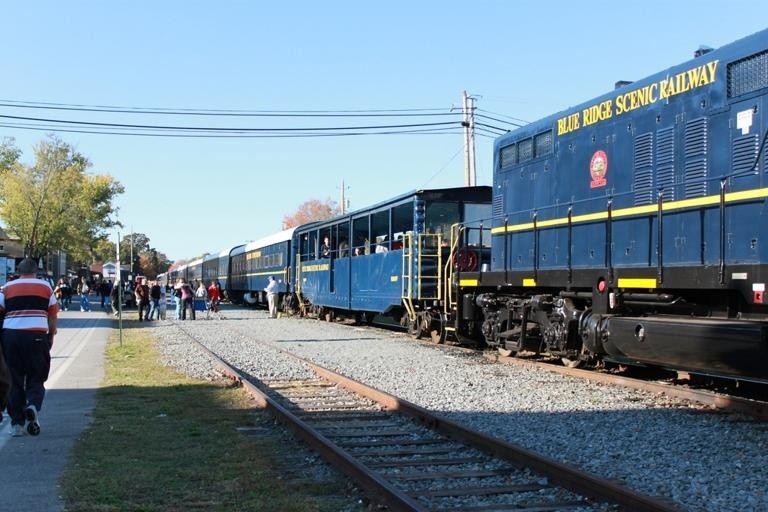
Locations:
153,27,766,382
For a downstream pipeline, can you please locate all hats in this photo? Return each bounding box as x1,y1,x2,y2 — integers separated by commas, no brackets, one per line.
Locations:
267,275,275,280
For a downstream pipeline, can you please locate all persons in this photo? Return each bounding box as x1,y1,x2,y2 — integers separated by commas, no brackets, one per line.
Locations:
1,304,15,422
319,224,451,258
53,271,231,325
263,275,280,320
1,259,58,437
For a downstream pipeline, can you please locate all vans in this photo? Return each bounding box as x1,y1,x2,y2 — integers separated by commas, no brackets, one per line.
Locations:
7,272,55,292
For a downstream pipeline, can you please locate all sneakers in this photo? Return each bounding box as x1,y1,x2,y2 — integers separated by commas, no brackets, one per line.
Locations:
23,405,41,435
7,424,23,436
139,315,225,322
58,301,119,318
268,315,277,319
